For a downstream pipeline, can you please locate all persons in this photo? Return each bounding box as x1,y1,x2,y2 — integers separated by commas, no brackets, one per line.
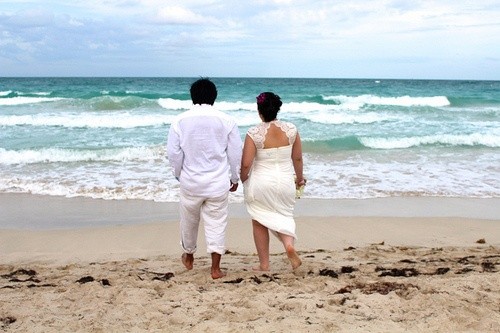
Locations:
239,92,307,271
168,78,243,278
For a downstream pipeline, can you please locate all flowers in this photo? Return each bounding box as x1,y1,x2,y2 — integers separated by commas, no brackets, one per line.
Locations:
256,94,268,110
